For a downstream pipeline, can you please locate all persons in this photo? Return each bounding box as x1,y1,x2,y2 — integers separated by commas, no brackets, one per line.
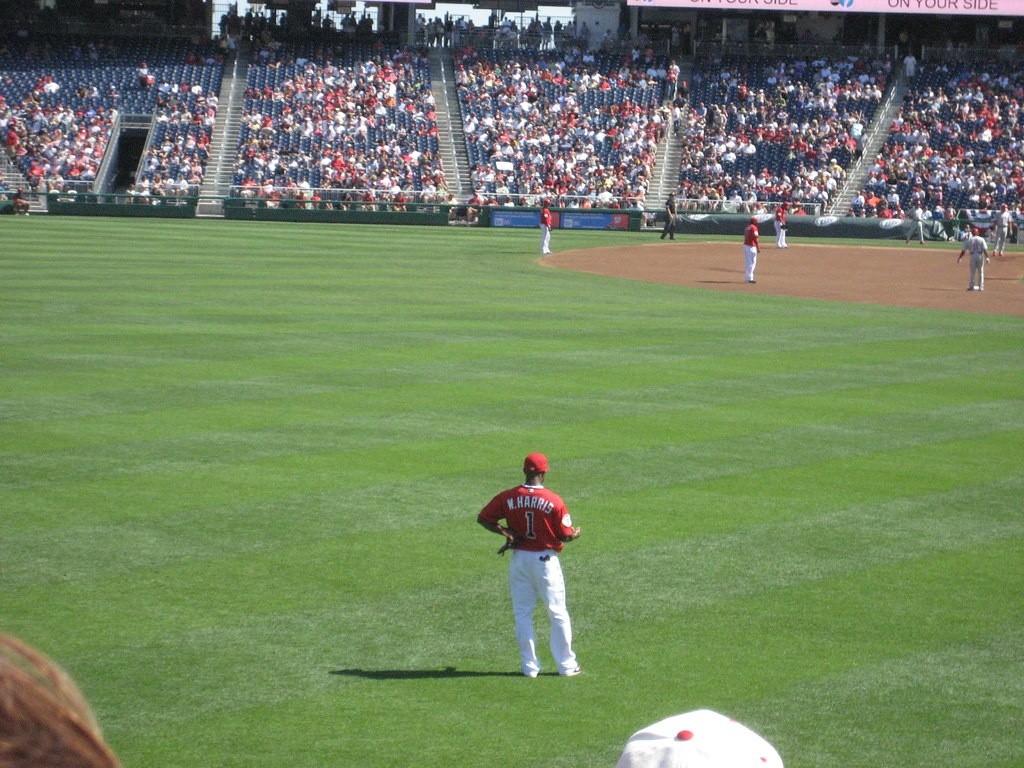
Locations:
452,15,693,229
743,217,759,284
540,200,552,255
615,709,783,768
231,6,453,211
0,7,239,201
993,204,1012,256
14,188,29,216
477,455,582,676
957,228,990,291
845,36,1024,217
0,634,120,768
660,193,677,240
774,202,789,248
905,201,924,244
675,18,920,213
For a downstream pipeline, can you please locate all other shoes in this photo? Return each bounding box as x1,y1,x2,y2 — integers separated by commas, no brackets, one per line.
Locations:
527,674,536,677
560,667,581,676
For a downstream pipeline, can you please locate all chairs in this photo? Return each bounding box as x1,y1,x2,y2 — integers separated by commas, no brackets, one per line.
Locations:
448,42,666,209
675,50,1024,220
227,37,445,212
2,34,226,202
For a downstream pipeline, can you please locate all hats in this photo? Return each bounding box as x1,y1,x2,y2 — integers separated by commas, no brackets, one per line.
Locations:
972,228,979,234
783,202,790,210
523,453,549,474
544,199,551,205
1001,204,1008,209
750,217,759,224
614,710,784,768
